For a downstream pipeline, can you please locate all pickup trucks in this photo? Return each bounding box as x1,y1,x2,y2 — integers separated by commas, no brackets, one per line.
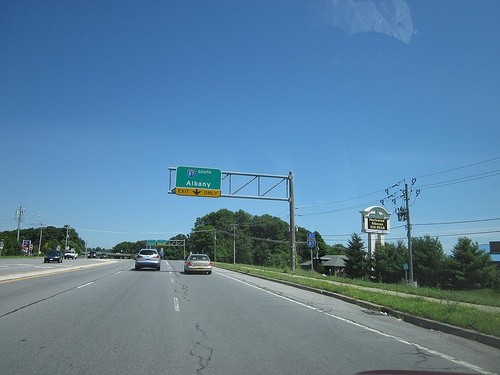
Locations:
65,250,78,260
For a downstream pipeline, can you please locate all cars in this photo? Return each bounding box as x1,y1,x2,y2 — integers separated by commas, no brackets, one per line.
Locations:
184,254,212,274
44,251,63,263
100,253,107,259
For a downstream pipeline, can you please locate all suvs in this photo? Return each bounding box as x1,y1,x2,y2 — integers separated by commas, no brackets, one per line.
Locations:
86,251,97,259
135,249,161,270
